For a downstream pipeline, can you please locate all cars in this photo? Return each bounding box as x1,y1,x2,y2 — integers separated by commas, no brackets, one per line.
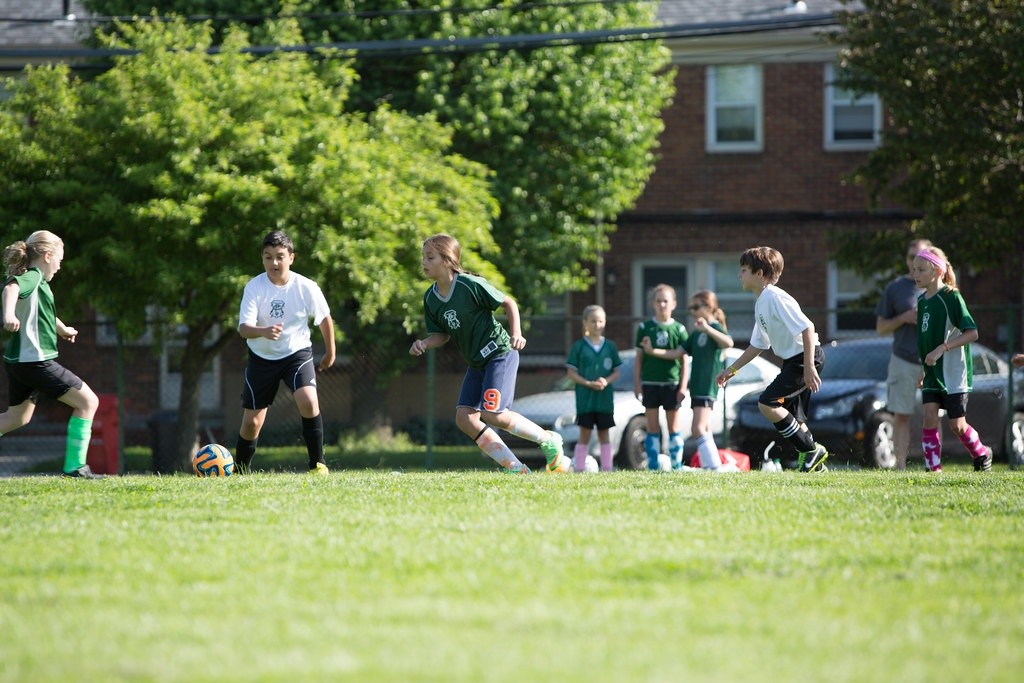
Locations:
482,347,783,473
727,336,1024,472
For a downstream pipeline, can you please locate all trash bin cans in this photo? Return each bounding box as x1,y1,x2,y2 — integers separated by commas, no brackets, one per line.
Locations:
146,409,227,474
86,393,130,474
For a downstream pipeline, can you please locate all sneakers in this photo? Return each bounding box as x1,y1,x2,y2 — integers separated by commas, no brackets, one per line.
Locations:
502,464,532,475
794,441,829,475
809,463,830,476
538,430,563,470
972,446,993,473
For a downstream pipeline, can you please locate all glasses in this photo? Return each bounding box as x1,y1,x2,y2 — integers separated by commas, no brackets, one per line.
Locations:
906,252,917,261
687,303,710,312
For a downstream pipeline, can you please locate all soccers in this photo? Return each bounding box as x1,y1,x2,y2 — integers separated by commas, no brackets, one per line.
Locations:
192,443,235,477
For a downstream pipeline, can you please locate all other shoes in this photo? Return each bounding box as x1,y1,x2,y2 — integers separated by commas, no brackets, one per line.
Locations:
60,465,108,481
308,462,329,481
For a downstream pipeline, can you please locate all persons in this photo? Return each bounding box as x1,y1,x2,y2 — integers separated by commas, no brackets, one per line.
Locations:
0,230,104,480
911,246,992,473
639,290,734,470
715,248,829,476
872,235,945,469
408,235,563,475
563,305,623,473
634,283,689,471
232,231,337,476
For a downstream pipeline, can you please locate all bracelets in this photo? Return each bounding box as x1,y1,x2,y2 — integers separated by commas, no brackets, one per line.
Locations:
729,366,736,374
944,342,950,351
